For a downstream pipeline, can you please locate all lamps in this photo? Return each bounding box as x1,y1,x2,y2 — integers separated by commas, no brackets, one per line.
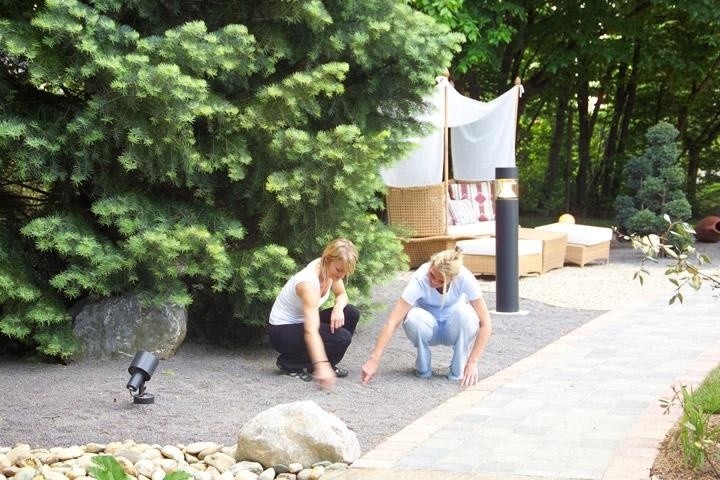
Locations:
124,348,163,406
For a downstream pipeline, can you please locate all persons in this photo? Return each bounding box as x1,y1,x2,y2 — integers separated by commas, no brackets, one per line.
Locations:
268,237,360,383
360,246,492,388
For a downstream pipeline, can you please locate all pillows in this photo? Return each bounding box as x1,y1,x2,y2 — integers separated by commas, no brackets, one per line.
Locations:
447,199,479,226
449,182,497,223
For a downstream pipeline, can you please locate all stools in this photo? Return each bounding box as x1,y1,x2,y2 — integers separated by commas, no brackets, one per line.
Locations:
455,237,543,280
534,223,614,268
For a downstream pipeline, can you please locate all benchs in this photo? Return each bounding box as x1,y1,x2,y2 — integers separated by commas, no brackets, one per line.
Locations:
384,175,521,274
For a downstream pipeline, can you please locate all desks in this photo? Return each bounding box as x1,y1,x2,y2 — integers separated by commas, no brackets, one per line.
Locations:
518,227,569,275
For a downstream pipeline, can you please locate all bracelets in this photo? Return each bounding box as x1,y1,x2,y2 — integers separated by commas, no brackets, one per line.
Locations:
312,360,331,365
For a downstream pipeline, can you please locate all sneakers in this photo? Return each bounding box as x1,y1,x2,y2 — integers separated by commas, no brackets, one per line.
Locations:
276,359,312,382
447,365,465,382
332,365,350,377
415,362,433,379
